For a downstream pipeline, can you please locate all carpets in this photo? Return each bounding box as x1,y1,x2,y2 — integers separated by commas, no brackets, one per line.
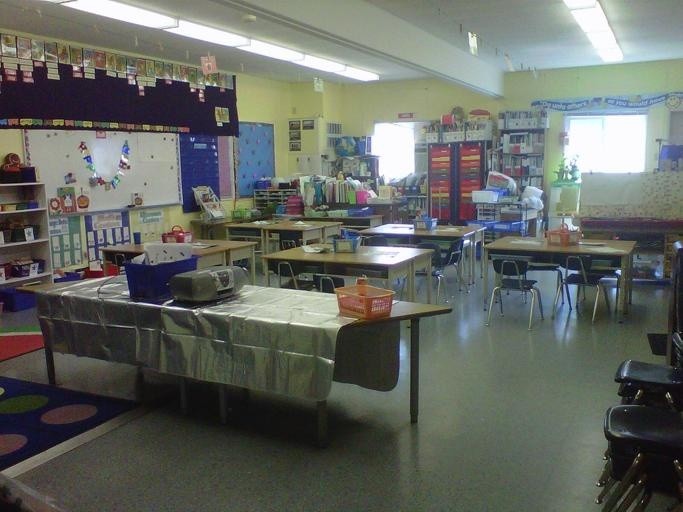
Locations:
0,371,150,502
0,287,74,362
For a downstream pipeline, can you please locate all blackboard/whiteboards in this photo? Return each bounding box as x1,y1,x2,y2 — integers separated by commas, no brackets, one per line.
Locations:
22,127,184,216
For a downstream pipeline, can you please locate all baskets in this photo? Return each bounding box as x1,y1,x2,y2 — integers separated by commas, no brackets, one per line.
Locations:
334,284,396,320
12,259,45,277
11,224,40,242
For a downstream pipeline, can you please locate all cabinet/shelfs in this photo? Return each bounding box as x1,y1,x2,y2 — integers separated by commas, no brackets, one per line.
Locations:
0,179,54,317
498,126,546,188
254,188,298,211
427,142,487,222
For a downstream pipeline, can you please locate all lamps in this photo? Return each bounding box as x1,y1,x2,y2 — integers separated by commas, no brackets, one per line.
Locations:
52,0,381,89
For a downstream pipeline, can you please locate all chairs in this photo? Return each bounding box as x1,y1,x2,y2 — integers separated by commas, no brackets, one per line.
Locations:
477,219,671,333
115,185,488,334
94,185,678,336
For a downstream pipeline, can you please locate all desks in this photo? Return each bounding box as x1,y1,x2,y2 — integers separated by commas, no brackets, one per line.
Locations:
16,270,455,451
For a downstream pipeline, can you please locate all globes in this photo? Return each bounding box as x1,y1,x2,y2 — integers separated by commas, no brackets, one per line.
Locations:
335,136,357,156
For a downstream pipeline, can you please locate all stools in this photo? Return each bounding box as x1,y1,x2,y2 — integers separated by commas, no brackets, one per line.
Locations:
594,358,682,511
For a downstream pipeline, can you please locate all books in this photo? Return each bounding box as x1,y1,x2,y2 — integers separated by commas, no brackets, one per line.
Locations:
193,185,225,219
427,111,547,220
310,175,354,203
130,243,193,265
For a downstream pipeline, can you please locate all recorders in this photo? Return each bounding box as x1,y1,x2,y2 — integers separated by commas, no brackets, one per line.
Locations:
169,266,246,307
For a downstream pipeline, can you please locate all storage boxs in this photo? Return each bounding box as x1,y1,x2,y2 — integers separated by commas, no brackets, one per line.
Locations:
498,117,553,130
1,281,47,314
83,263,119,279
53,269,85,284
0,199,45,282
426,122,493,142
